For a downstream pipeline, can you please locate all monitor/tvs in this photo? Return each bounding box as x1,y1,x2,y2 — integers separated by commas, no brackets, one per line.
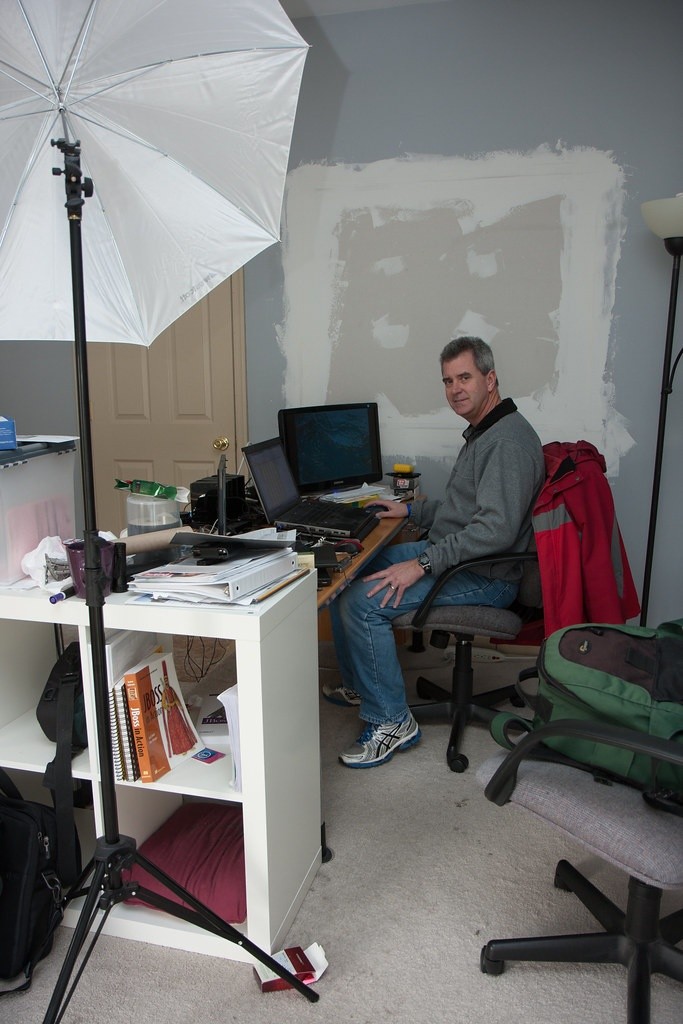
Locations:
278,403,383,496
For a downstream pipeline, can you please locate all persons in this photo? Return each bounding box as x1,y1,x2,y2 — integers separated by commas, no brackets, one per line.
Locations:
322,338,546,769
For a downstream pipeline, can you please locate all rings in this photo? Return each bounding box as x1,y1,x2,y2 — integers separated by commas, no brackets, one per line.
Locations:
390,586,396,591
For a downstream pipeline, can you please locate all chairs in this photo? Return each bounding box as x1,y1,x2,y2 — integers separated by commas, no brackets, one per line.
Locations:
409,446,595,772
476,730,683,1024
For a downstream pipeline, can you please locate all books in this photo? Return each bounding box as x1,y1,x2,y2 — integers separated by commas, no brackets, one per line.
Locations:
106,630,206,782
218,684,242,791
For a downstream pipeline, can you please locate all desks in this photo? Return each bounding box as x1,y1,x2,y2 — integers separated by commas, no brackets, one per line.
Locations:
315,496,425,641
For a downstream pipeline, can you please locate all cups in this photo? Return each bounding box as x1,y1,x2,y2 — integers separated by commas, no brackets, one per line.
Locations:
66,540,117,597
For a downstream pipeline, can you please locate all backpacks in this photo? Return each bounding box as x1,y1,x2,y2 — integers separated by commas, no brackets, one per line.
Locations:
485,618,683,814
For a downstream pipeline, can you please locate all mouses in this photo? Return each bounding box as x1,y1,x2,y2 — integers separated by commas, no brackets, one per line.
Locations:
364,504,389,514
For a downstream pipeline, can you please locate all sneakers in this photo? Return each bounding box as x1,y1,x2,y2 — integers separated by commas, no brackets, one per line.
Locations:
322,682,362,706
338,707,422,768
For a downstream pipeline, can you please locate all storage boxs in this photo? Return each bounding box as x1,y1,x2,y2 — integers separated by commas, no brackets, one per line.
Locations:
1,440,76,588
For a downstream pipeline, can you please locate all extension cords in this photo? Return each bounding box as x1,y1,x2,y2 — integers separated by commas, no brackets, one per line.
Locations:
444,646,505,662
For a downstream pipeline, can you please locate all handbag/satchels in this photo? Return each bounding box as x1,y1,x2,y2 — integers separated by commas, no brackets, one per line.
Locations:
36,642,90,746
0,766,63,999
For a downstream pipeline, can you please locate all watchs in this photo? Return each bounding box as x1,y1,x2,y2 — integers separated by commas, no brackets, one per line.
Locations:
418,553,432,574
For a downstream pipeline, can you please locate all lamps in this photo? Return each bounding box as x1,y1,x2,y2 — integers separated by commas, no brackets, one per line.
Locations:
641,191,683,625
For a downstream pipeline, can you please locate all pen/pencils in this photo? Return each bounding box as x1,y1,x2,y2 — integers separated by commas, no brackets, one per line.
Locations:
49,586,78,605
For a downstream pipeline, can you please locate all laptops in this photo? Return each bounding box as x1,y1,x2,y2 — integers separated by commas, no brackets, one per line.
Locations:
241,436,376,537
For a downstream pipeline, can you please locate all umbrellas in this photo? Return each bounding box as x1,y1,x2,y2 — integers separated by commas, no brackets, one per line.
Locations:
1,0,316,347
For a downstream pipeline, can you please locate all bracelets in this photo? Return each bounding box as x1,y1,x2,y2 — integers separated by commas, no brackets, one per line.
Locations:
406,503,412,516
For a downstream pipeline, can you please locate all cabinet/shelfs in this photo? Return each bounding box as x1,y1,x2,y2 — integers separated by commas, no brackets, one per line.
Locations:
1,558,323,964
